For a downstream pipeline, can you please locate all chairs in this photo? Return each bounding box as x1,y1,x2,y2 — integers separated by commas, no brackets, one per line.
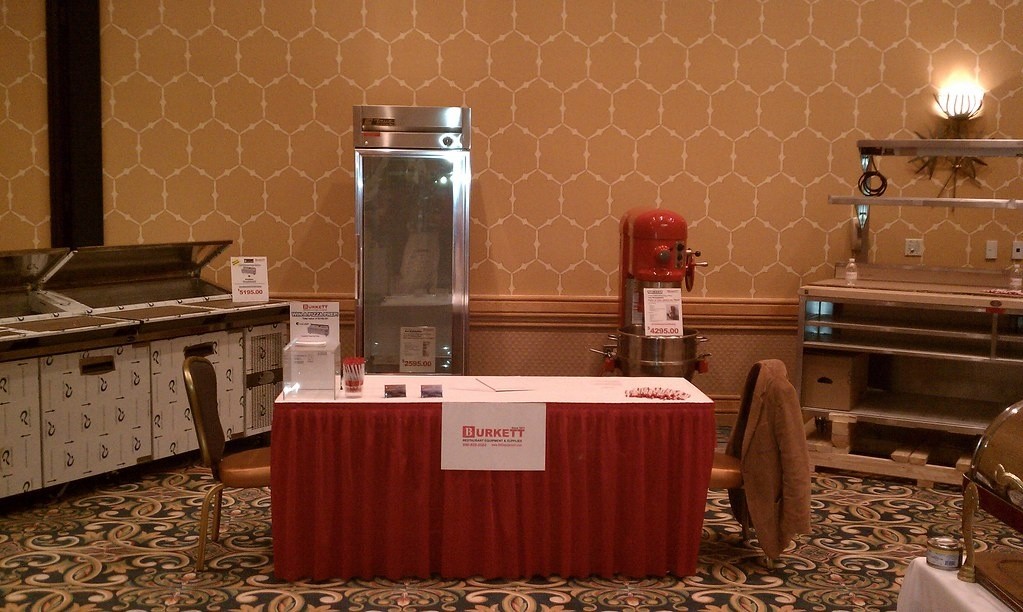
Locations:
182,353,270,574
708,359,807,567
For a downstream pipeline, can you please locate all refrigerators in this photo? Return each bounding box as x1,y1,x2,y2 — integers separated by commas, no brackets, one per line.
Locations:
352,105,472,376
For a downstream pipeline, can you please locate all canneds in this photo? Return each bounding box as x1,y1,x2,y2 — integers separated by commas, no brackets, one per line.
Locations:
926,537,963,571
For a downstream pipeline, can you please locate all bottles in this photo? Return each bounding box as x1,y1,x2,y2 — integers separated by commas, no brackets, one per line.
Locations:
1010,262,1022,291
845,258,857,286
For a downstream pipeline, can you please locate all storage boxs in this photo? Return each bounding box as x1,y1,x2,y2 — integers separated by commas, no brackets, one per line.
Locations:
803,353,870,410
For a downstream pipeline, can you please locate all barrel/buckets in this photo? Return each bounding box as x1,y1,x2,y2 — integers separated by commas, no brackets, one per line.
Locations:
614,325,699,385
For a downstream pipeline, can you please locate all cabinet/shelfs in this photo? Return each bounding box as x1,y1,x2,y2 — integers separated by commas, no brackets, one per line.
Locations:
1,320,286,503
795,279,1023,488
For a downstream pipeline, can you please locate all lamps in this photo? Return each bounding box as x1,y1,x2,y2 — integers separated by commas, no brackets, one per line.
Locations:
931,65,988,139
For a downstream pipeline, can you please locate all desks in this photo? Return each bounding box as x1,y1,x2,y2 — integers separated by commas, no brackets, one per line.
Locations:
896,553,1014,611
271,376,716,583
